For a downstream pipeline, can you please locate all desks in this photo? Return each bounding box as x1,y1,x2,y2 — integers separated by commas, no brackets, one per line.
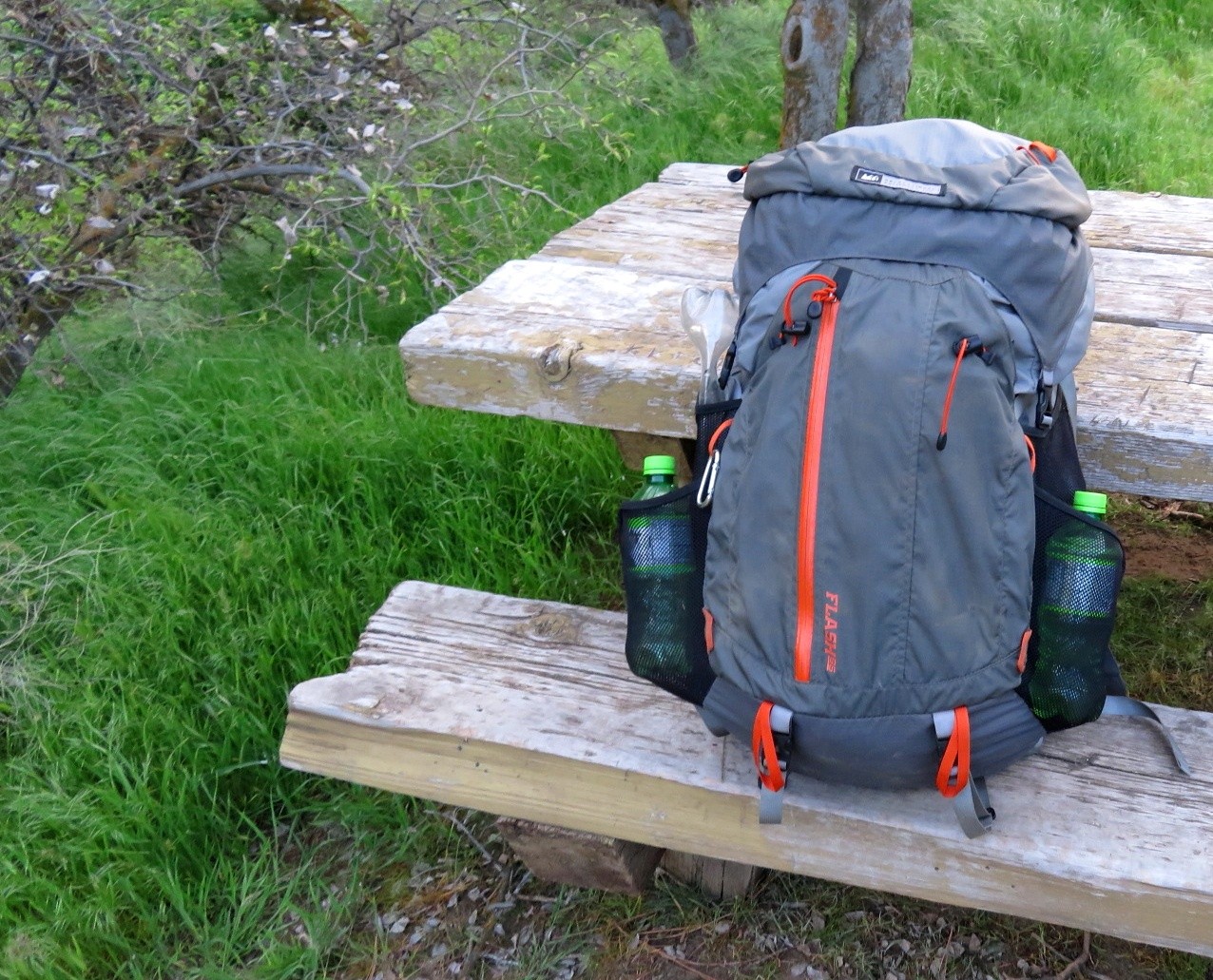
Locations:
399,162,1213,503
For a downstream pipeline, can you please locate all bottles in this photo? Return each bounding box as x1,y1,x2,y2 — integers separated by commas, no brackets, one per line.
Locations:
621,455,696,679
1023,491,1125,725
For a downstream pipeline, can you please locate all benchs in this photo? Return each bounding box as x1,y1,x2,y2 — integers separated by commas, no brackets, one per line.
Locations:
278,578,1213,962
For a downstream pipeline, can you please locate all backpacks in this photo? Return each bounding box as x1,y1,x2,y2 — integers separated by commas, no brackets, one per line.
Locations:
617,117,1189,839
